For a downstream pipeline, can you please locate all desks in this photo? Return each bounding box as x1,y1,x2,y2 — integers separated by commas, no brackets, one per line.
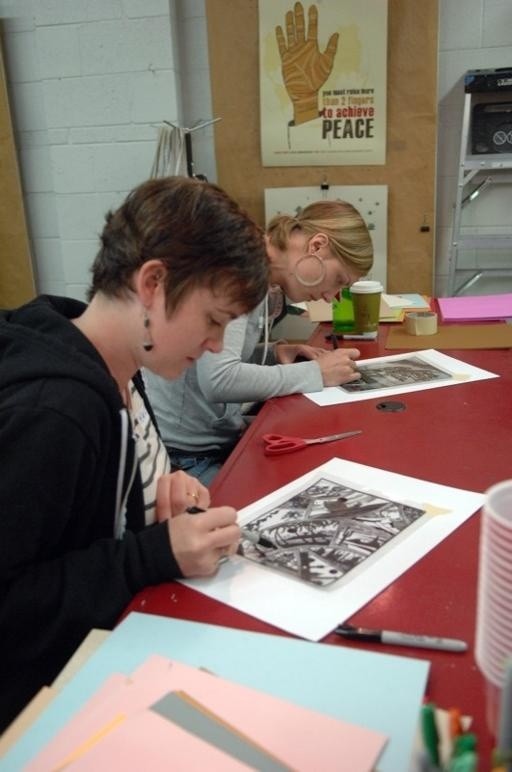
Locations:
115,319,509,772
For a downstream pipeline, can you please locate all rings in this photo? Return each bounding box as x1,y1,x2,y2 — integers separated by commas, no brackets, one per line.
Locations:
187,491,201,504
217,556,229,564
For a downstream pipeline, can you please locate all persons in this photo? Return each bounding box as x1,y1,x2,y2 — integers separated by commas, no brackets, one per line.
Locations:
274,2,340,150
120,197,375,486
1,171,277,739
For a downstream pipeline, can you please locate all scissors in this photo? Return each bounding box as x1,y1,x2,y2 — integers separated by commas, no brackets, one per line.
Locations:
262,430,362,456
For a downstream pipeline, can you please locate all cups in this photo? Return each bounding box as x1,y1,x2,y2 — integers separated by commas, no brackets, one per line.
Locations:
331,280,383,339
475,478,511,737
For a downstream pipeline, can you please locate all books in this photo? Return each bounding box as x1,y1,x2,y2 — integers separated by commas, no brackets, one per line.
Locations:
0,609,433,772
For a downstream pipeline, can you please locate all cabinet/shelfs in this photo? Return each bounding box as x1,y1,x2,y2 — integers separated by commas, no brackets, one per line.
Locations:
433,94,512,298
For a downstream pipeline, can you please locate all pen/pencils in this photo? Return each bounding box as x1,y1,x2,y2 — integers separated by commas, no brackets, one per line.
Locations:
335,625,467,653
185,505,279,551
330,332,338,350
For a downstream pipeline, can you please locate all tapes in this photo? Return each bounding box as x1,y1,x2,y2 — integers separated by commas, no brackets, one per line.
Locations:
404,312,438,335
